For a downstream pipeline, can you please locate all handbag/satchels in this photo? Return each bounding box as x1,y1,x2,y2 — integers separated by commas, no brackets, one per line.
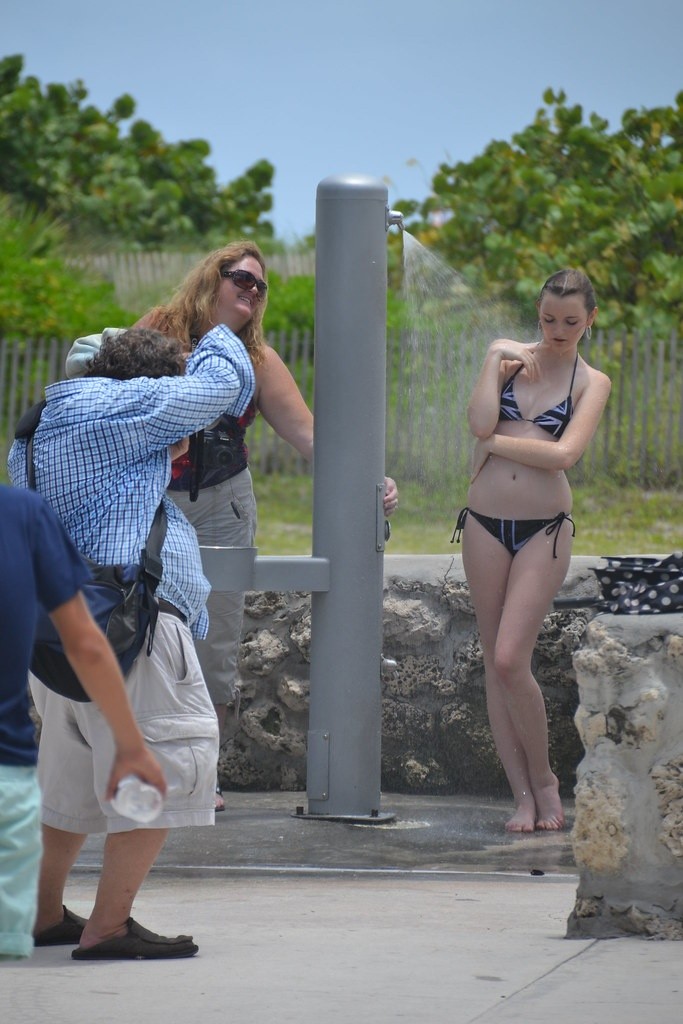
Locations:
28,552,162,703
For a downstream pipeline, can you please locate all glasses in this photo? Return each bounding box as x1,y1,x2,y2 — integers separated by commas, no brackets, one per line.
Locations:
221,269,268,298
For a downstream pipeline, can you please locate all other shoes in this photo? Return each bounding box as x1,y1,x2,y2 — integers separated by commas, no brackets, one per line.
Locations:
28,904,89,946
213,778,224,812
69,916,198,962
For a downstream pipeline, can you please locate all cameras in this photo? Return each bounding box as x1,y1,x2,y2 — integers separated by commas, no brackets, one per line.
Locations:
199,430,238,468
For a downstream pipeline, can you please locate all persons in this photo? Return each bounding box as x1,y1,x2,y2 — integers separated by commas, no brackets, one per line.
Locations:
4,328,256,961
129,242,400,809
450,268,611,832
0,487,168,957
